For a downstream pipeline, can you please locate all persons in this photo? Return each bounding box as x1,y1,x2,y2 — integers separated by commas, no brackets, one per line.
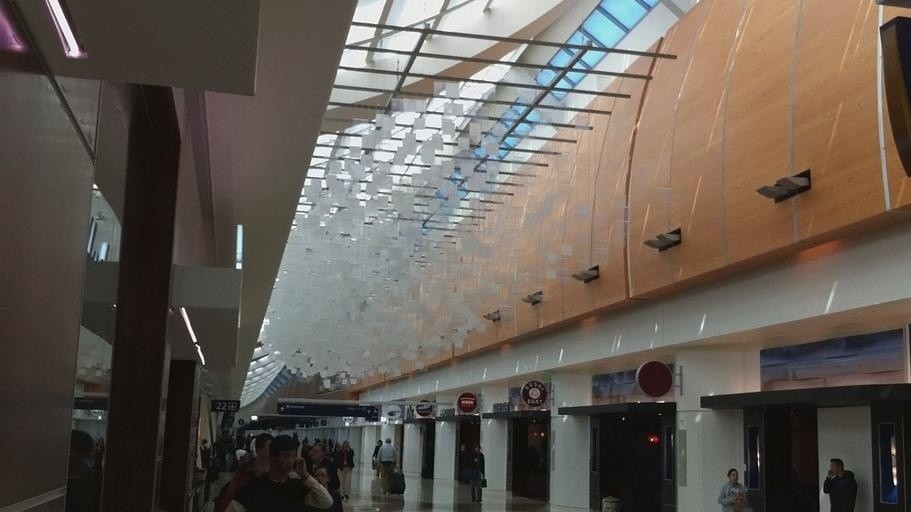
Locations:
823,458,858,512
467,444,486,502
717,468,748,512
372,438,397,495
200,432,355,512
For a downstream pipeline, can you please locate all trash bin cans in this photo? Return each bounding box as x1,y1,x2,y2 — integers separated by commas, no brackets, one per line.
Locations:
602,496,621,512
192,467,207,512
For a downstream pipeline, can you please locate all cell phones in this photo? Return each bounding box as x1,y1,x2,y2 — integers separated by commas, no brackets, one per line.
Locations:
294,460,299,470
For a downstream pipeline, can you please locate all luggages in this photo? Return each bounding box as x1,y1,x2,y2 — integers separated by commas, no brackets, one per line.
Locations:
386,465,405,494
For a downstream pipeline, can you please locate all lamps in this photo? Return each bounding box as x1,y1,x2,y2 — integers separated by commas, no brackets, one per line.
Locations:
642,227,681,253
571,265,599,284
482,291,543,322
753,169,812,203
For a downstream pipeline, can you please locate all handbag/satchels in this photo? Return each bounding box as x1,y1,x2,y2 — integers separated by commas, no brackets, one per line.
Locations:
481,478,487,487
